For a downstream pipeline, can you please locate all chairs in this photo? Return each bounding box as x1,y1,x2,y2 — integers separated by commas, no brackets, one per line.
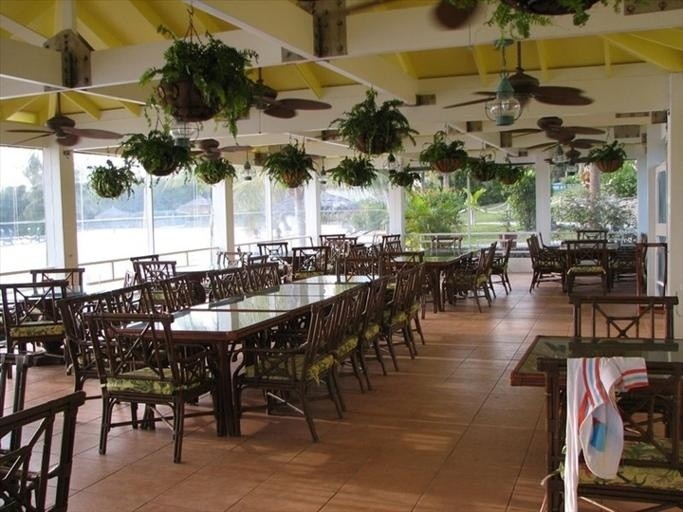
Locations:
564,292,680,443
54,291,155,431
532,350,682,512
282,281,370,411
0,351,31,459
1,232,525,390
523,225,648,298
82,311,226,463
0,387,85,511
232,292,348,443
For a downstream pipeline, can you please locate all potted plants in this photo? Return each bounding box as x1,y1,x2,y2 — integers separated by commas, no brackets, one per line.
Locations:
190,151,239,189
85,155,140,201
116,126,198,182
260,138,316,189
138,12,260,138
329,87,418,158
326,129,525,191
581,139,626,172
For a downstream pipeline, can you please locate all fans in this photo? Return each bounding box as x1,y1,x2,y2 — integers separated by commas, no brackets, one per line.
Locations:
505,112,606,166
445,37,592,109
4,92,123,148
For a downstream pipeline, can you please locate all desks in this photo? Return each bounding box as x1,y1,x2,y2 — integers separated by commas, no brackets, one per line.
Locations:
509,333,682,511
120,311,287,436
